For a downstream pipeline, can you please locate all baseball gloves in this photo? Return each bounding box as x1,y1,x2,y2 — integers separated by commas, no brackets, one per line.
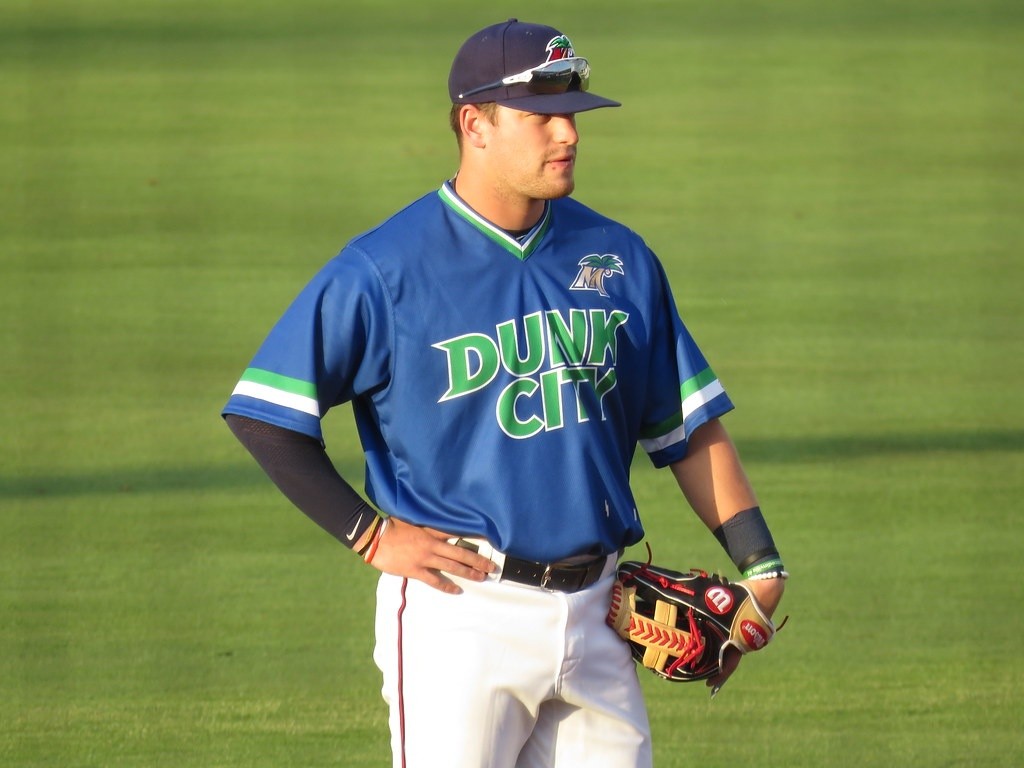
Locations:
604,559,777,685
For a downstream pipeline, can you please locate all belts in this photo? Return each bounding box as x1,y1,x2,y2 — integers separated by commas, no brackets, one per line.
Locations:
456,538,625,595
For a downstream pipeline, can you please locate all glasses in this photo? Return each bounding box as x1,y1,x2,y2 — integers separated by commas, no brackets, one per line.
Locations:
458,56,592,99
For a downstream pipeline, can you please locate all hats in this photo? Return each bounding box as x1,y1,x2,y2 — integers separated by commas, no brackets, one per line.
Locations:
448,17,622,114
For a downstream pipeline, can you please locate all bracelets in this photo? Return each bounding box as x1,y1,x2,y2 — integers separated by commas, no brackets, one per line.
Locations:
355,515,386,566
713,508,789,581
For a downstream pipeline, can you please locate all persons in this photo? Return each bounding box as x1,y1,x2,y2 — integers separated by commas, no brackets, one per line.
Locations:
221,18,787,767
